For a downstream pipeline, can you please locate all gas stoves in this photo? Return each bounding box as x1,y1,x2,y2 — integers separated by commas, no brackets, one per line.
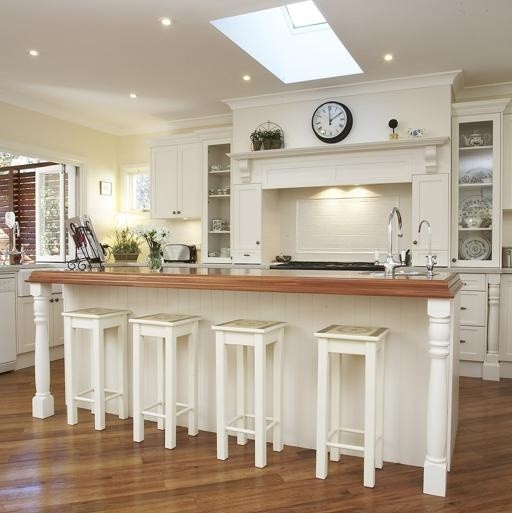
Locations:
270,260,386,271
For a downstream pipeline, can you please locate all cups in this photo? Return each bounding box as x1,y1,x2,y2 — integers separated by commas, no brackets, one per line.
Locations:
211,218,226,230
219,246,230,257
209,186,230,195
503,246,512,267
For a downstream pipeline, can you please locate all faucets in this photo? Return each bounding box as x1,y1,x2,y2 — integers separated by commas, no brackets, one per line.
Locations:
417,219,434,257
386,206,403,257
11,221,21,252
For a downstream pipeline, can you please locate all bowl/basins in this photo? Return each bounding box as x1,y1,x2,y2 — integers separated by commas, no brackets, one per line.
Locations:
463,217,483,227
276,255,291,262
481,177,492,183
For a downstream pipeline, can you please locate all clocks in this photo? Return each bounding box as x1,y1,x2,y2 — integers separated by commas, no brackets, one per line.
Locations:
311,101,353,144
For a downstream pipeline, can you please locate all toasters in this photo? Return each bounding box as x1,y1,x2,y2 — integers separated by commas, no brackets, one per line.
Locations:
162,243,198,262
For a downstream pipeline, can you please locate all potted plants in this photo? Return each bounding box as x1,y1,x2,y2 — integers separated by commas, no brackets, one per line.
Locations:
249,129,281,151
104,224,146,266
139,224,170,273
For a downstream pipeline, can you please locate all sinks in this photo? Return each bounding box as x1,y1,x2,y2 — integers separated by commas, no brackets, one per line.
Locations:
358,269,438,277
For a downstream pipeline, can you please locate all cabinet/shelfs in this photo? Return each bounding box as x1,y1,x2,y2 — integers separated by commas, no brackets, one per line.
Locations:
456,273,488,378
411,174,451,267
150,139,202,220
202,137,233,265
233,183,281,265
16,267,64,370
451,98,511,269
0,266,17,374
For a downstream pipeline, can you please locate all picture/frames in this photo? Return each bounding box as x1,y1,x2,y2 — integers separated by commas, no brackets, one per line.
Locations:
100,181,112,196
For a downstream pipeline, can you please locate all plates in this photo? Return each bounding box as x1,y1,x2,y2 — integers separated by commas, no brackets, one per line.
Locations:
458,160,492,183
458,232,492,260
458,194,492,227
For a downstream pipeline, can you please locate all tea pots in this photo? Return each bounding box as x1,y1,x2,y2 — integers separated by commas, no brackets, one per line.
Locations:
461,128,490,145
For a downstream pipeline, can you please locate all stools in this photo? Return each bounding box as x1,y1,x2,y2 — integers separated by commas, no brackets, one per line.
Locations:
313,324,390,488
61,308,132,431
211,319,289,468
129,312,203,450
